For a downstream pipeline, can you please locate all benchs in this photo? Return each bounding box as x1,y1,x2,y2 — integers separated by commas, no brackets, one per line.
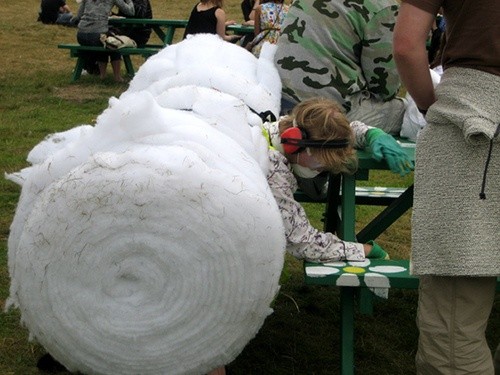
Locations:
57,43,164,57
294,187,408,205
302,259,500,290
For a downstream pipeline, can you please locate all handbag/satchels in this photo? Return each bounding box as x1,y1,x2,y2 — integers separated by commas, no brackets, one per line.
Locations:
105,26,137,50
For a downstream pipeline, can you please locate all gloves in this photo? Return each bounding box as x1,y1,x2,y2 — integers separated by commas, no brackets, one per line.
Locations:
365,240,390,259
366,128,415,177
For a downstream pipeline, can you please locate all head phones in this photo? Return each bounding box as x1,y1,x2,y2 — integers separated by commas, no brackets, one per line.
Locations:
280,127,349,154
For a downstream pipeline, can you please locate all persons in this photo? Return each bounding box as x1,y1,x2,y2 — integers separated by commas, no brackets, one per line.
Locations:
239,0,284,47
393,0,500,375
183,0,242,42
76,0,135,83
40,0,78,27
274,0,409,135
108,0,153,47
263,96,390,263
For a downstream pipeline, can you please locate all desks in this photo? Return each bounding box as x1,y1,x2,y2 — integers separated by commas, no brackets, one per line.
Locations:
287,137,417,374
227,24,254,47
70,16,191,83
